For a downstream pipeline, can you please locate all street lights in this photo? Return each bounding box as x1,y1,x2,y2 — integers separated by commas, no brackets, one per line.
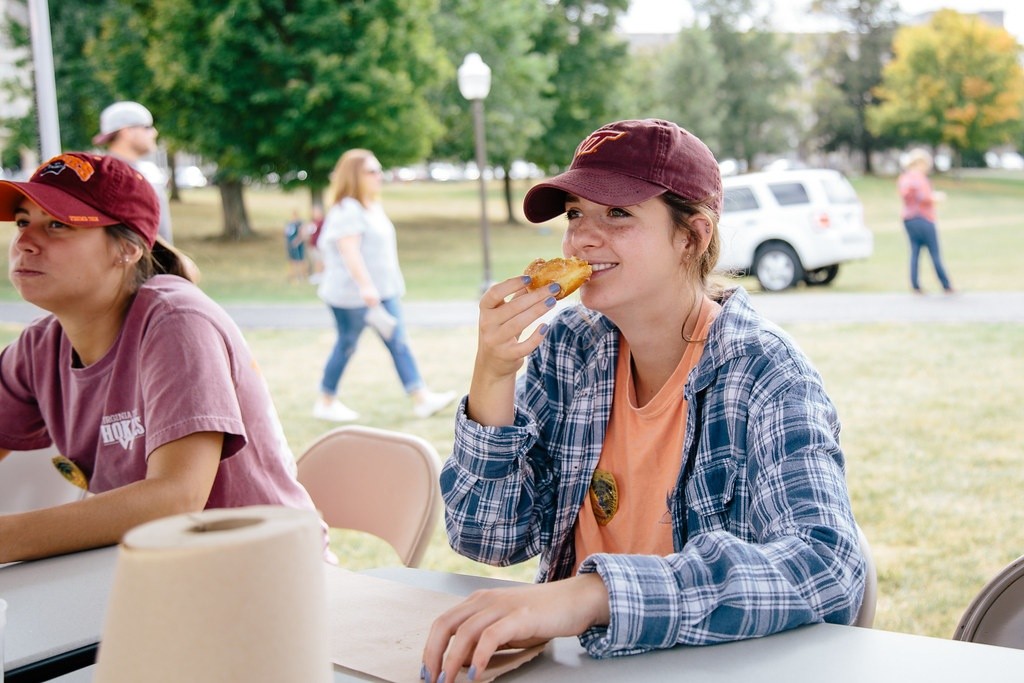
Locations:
458,53,494,291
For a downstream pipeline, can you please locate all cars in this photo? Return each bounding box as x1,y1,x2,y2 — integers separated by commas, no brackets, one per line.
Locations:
719,168,873,292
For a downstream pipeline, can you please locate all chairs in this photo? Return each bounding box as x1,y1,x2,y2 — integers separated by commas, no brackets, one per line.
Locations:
851,521,879,628
952,554,1024,651
296,425,444,568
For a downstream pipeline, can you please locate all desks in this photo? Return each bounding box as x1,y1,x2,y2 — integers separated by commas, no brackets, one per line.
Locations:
19,559,1024,682
0,442,238,682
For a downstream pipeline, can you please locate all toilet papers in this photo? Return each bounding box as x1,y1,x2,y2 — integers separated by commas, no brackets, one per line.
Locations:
90,501,333,683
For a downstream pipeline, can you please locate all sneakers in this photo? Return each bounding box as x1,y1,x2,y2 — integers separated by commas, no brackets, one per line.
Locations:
312,398,362,421
413,390,459,419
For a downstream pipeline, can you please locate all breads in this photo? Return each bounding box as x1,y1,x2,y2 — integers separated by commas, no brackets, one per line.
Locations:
522,258,592,300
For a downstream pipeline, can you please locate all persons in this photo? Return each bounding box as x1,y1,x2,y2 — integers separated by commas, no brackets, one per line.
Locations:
0,151,333,572
418,116,868,683
91,100,173,248
307,147,459,418
894,146,957,295
282,204,328,282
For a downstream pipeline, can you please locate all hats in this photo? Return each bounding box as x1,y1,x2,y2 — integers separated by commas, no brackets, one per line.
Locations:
91,101,154,145
1,151,162,251
524,118,723,224
